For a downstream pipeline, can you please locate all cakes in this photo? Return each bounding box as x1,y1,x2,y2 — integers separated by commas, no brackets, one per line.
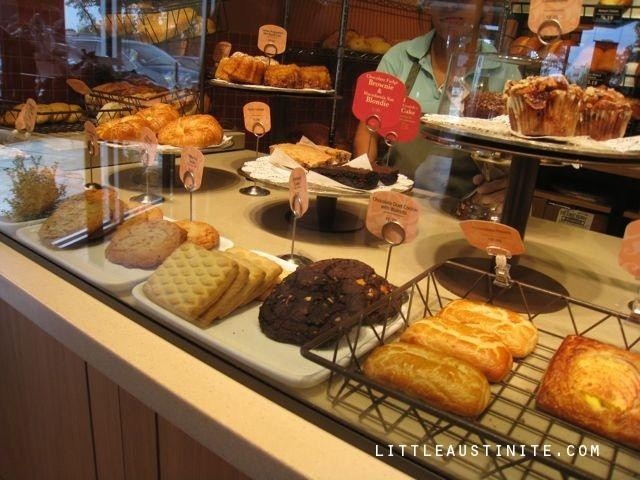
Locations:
502,73,633,140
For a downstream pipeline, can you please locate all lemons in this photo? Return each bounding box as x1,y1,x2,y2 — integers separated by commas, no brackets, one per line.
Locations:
348,40,391,55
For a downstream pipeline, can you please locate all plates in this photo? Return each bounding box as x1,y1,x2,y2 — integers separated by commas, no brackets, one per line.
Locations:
0,199,52,240
129,247,407,389
16,212,233,296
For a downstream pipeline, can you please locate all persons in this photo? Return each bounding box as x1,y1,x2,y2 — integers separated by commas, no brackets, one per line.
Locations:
353,0,525,215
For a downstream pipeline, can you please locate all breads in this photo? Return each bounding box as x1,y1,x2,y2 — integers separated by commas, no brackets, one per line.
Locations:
375,165,398,186
510,33,541,58
104,222,189,269
365,299,536,418
461,89,507,119
258,259,408,348
92,103,224,148
314,165,378,190
0,99,83,131
269,143,350,170
176,220,218,249
42,189,163,243
534,335,640,450
94,2,214,43
542,40,576,62
84,80,198,114
214,50,333,89
139,245,288,327
322,31,385,52
